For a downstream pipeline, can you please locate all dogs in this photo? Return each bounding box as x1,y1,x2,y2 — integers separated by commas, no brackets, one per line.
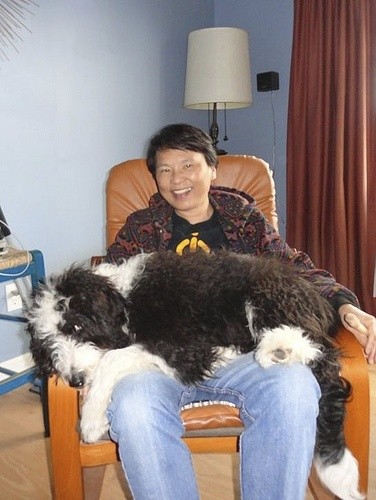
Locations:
21,248,368,500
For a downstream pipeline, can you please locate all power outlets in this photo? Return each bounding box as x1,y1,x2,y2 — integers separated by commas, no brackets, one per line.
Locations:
5,283,23,311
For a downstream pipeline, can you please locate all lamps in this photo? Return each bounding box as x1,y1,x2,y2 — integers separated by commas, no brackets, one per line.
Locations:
182,26,254,156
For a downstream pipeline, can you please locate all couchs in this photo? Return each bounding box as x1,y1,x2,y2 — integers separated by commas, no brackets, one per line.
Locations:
48,153,376,500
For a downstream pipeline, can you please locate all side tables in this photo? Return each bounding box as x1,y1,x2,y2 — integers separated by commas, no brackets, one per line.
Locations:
0,248,52,439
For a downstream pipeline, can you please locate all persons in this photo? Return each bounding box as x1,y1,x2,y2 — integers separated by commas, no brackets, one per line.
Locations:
101,123,376,500
